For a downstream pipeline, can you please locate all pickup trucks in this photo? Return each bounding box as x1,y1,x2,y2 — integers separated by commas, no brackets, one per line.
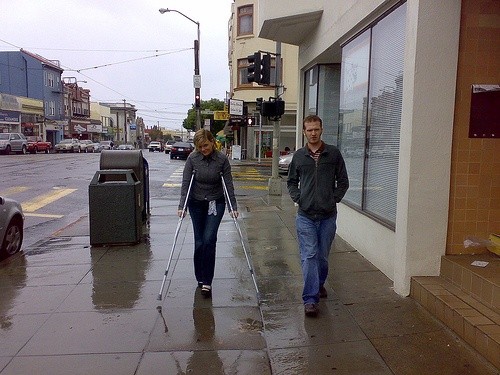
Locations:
26,136,52,154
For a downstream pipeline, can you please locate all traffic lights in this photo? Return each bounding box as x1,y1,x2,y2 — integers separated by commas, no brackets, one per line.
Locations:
255,97,263,110
260,55,271,84
247,52,261,82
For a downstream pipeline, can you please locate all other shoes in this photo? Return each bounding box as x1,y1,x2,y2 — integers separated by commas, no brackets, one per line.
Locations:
305,304,318,314
201,285,212,296
319,286,327,297
198,281,203,287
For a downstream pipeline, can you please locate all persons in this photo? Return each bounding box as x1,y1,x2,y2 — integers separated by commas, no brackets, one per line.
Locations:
177,130,239,297
110,142,114,150
285,115,350,316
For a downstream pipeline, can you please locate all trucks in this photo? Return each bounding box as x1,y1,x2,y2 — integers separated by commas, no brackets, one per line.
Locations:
147,141,164,152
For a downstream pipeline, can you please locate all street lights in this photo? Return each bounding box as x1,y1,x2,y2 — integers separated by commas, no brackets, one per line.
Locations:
159,8,201,131
67,80,87,134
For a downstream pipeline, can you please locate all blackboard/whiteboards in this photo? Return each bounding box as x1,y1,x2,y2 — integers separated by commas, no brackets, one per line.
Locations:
232,145,241,158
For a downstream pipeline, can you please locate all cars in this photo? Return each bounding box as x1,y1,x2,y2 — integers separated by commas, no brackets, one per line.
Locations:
279,153,294,171
0,196,25,255
116,145,135,150
55,138,115,153
170,142,194,159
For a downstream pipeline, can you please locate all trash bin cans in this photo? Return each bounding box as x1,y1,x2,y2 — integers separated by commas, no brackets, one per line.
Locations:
99,148,151,225
88,168,144,245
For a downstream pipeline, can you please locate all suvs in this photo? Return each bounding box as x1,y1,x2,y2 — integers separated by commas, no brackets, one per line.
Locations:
164,141,176,154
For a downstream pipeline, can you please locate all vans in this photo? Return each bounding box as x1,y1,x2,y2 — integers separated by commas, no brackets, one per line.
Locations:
0,133,28,154
